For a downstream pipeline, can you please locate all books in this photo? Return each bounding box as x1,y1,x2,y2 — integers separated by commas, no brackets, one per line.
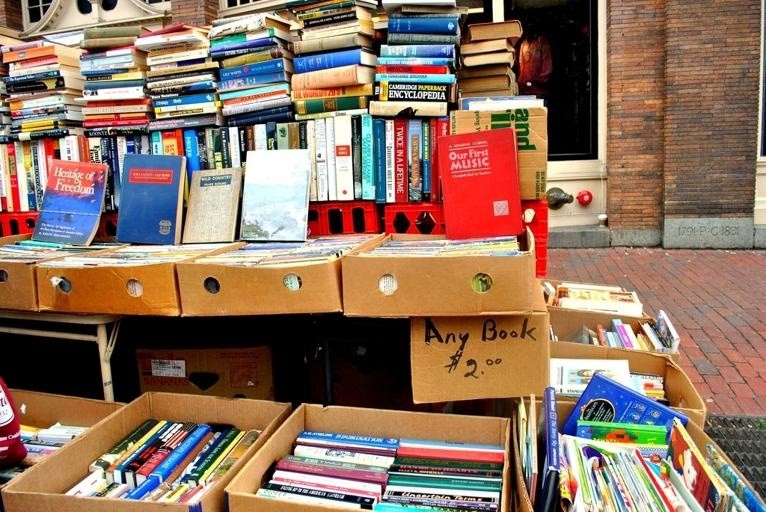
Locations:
1,2,766,511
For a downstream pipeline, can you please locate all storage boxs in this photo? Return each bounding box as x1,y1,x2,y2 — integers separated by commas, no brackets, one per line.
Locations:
225,402,510,512
137,347,274,401
410,278,550,404
0,234,129,312
3,391,291,512
342,225,535,318
176,232,385,317
0,389,128,493
38,242,246,317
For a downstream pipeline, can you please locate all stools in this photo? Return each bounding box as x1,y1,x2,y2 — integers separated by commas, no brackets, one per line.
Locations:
0,309,124,402
